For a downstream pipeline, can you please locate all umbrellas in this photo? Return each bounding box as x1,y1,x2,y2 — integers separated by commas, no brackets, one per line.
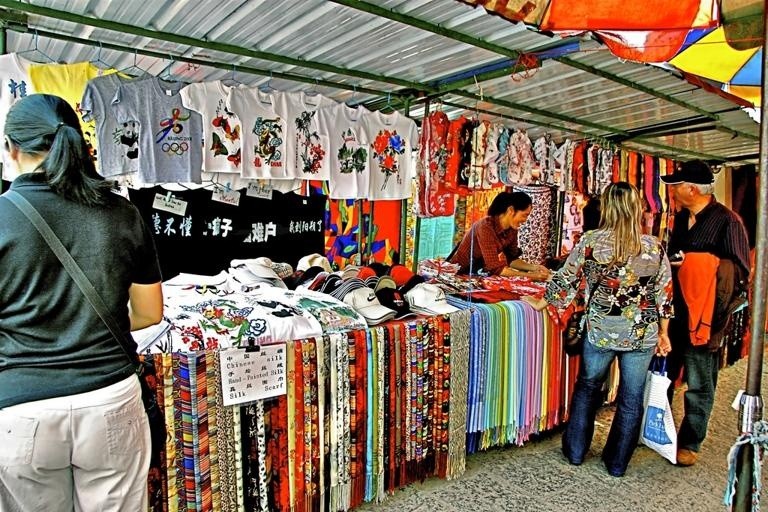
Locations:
459,0,768,512
644,20,763,108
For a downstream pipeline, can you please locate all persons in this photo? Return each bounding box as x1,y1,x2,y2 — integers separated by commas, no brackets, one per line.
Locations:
637,159,751,467
450,192,551,282
520,182,675,477
0,94,163,512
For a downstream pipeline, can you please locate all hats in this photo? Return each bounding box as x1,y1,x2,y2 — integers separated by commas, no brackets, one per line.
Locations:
658,158,715,184
231,253,461,326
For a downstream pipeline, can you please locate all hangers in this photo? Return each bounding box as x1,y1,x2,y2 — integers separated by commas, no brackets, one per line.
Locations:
16,29,57,65
379,92,394,114
304,77,321,96
345,84,359,108
219,64,277,93
88,40,178,82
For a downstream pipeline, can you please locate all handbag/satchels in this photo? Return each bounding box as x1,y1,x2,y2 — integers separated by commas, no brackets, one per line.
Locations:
637,370,677,465
563,310,587,355
141,386,167,453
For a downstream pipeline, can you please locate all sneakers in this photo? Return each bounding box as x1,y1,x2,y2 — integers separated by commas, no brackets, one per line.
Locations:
677,447,698,465
